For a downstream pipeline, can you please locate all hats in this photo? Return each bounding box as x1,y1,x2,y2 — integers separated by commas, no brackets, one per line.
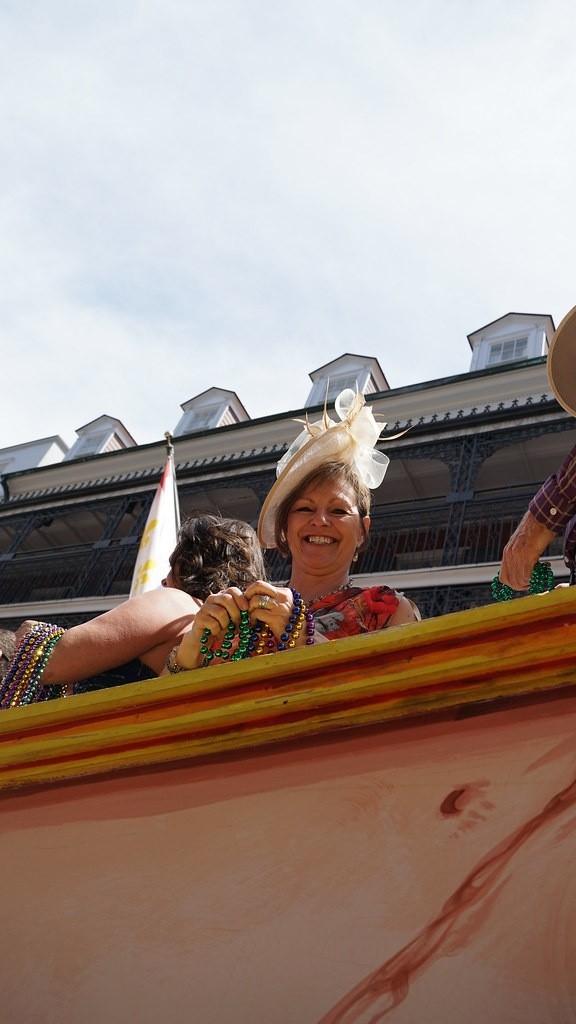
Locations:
548,305,576,417
258,376,420,549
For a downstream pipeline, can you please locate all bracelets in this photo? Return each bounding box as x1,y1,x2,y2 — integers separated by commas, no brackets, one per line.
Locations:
165,647,184,674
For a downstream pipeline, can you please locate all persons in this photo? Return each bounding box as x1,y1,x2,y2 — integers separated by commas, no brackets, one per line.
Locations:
0,512,265,696
496,305,576,591
158,383,415,676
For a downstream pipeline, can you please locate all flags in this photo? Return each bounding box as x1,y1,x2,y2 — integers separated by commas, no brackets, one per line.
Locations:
128,456,176,600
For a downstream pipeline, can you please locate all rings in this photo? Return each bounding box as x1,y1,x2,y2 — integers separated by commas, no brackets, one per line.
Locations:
259,595,270,609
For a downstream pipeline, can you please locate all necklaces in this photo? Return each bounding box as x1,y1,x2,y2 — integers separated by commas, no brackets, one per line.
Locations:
490,565,553,601
305,577,354,608
198,587,315,663
0,622,67,709
